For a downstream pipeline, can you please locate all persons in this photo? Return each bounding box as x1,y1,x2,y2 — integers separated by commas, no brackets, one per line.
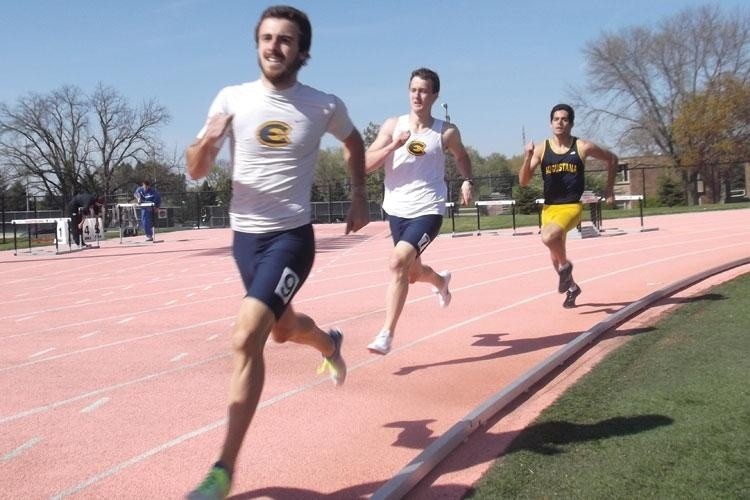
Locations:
67,192,106,249
133,179,161,242
365,68,476,356
182,4,373,499
518,103,618,309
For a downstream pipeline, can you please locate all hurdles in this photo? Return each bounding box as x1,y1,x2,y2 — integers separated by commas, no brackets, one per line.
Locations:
116,203,156,244
475,200,516,235
11,217,99,256
536,190,643,234
444,202,455,234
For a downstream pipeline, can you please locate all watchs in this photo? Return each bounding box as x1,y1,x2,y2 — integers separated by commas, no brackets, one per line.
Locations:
462,177,475,186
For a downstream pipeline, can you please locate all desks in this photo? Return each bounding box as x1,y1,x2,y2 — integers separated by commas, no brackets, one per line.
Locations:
79,217,105,251
11,218,72,256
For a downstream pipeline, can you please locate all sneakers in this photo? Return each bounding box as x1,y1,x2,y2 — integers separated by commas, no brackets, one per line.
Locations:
318,329,346,386
186,461,231,500
367,328,393,356
559,261,581,309
434,269,451,308
145,235,153,241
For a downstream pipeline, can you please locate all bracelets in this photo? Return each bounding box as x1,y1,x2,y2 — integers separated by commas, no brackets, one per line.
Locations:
606,185,614,190
